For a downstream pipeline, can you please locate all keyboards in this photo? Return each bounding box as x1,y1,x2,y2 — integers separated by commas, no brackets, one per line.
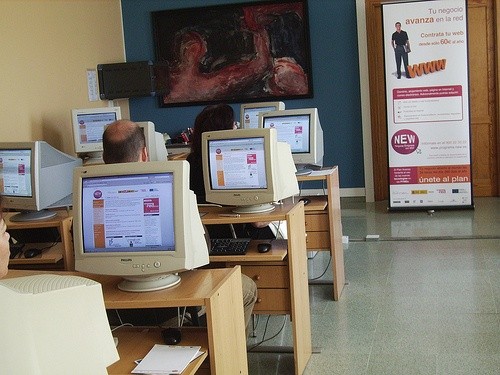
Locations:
9,242,24,259
210,238,250,256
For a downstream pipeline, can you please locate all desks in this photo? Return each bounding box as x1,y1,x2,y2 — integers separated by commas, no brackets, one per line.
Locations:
0,165,346,375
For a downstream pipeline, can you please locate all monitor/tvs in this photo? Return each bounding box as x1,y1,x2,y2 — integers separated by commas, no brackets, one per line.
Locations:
0,141,82,222
103,121,168,163
97,61,154,100
240,101,285,128
70,106,121,163
73,160,209,292
0,273,120,375
257,107,324,174
202,128,299,214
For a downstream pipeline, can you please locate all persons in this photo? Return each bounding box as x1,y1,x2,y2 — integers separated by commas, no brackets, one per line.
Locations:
0,193,11,280
186,104,273,241
103,119,258,342
392,22,412,79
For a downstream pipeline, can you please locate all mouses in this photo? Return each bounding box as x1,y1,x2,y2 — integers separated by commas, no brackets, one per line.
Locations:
257,242,271,253
24,248,42,258
162,327,181,344
299,198,311,206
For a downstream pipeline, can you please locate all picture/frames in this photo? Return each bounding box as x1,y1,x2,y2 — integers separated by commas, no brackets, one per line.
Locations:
151,0,313,108
